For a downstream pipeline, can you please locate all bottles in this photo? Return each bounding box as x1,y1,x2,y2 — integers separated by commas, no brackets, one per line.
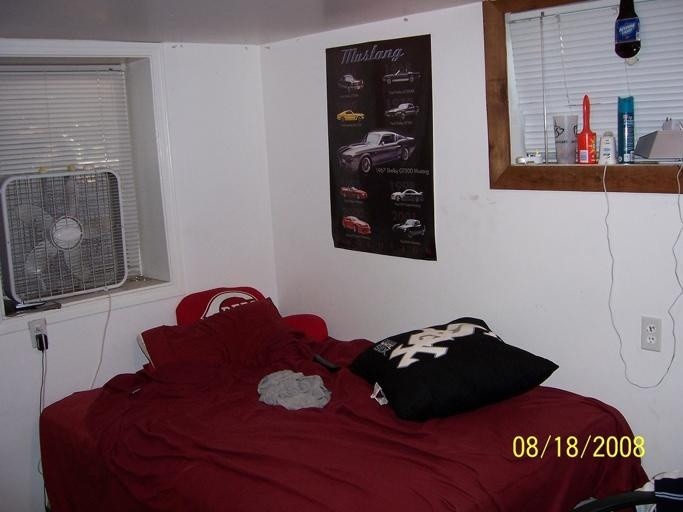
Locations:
615,1,641,59
616,95,635,164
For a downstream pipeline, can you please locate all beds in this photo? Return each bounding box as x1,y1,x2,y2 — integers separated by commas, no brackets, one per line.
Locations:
39,333,648,511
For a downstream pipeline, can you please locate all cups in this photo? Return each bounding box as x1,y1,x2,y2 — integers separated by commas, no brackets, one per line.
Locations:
553,116,578,165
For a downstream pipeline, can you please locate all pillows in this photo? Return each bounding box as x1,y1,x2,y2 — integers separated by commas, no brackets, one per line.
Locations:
283,314,329,345
175,287,266,323
138,297,283,387
348,316,560,422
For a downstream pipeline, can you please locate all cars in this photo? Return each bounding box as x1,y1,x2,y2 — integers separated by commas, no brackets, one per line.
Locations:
337,73,364,92
342,215,371,236
383,102,423,121
392,218,424,239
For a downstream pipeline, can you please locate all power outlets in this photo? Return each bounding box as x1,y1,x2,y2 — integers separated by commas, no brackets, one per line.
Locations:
640,316,662,353
28,318,48,349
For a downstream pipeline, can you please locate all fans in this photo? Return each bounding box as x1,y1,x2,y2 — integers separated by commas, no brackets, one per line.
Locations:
1,168,127,304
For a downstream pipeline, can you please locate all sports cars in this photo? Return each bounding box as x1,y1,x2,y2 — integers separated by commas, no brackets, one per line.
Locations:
390,188,424,205
339,184,367,201
334,130,416,176
383,67,421,84
336,109,368,122
